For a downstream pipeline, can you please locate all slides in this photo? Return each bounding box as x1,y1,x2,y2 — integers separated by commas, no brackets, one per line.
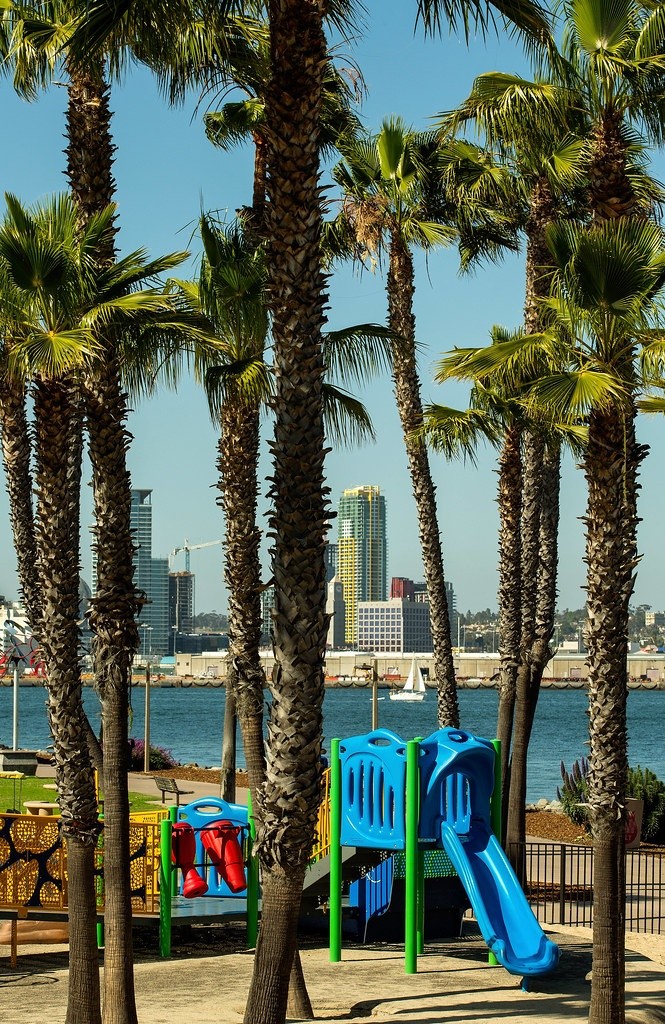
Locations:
439,820,558,978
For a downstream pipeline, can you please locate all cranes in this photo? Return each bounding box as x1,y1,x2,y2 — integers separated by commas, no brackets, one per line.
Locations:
173,539,227,572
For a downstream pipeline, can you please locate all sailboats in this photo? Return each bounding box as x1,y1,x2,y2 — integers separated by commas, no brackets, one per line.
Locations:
389,650,426,701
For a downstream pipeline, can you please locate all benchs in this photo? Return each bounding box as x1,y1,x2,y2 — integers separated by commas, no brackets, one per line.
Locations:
153,776,194,805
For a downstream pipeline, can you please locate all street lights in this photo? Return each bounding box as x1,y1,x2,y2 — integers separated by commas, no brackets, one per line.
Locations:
355,660,378,746
141,622,148,655
147,626,154,652
131,661,149,773
